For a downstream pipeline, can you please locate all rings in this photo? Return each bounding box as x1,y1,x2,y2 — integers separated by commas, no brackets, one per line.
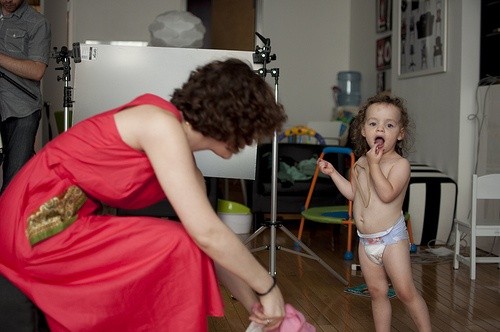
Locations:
267,319,271,325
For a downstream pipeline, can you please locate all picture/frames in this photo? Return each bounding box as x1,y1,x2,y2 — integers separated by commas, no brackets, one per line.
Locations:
375,35,393,71
375,0,393,34
396,0,448,79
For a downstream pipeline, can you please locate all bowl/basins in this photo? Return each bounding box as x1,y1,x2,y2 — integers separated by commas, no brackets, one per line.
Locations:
216,198,252,234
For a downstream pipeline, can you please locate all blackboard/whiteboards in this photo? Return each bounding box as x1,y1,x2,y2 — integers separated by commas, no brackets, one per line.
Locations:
71,44,263,180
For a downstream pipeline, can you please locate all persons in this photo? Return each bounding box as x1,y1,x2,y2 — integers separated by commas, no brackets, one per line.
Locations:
0,0,52,197
317,94,431,332
0,56,288,332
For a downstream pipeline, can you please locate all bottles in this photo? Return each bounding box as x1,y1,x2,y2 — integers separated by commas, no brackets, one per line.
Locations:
337,71,361,106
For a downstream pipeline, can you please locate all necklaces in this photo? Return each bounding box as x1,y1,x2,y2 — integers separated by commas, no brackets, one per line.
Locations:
186,121,189,136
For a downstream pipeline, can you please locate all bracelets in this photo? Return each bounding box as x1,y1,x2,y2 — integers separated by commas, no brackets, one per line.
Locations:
256,275,277,296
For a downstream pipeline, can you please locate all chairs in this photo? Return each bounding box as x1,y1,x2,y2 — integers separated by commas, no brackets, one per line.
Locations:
250,142,346,251
451,172,500,280
